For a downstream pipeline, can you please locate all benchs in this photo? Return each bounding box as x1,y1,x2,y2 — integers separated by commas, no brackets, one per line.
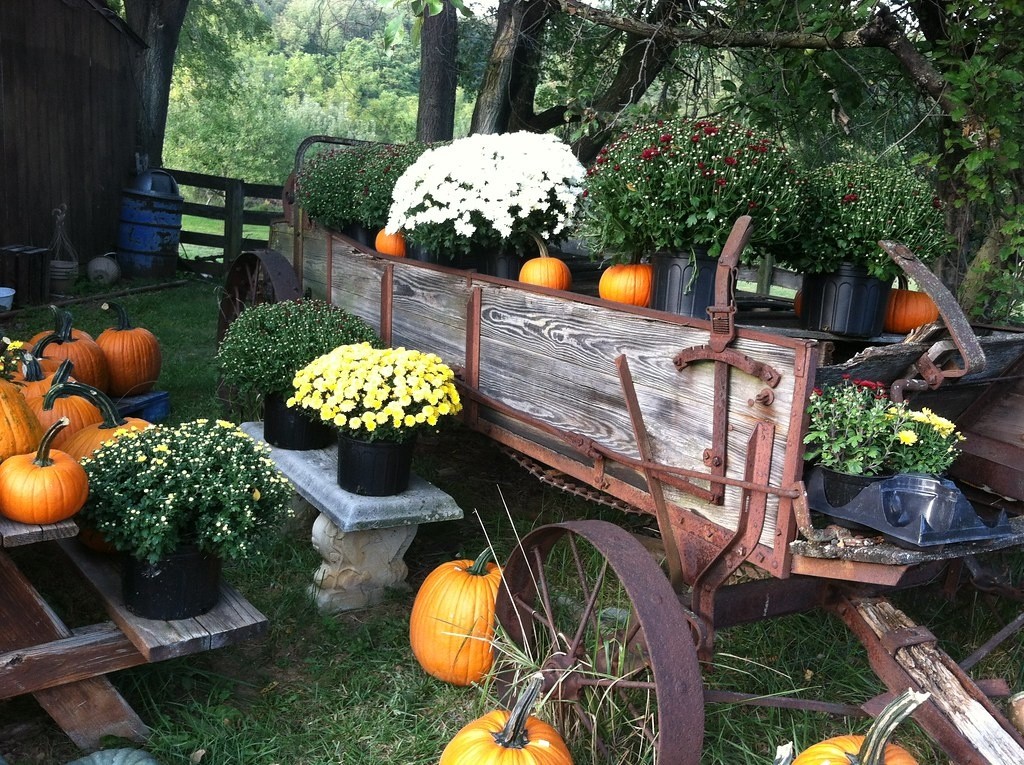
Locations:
238,422,465,615
57,540,268,662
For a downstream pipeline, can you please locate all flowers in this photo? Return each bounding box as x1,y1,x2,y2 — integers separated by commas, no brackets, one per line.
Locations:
77,418,295,577
287,342,464,444
215,299,386,402
294,119,952,281
886,406,967,476
801,372,909,478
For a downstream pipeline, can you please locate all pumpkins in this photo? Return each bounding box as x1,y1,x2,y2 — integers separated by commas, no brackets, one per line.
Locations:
438,671,575,765
410,545,509,684
519,230,571,291
794,288,802,320
375,228,406,256
886,274,938,334
790,688,931,765
0,302,161,553
599,251,653,307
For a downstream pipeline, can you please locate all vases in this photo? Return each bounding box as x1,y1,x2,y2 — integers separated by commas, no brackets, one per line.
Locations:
446,246,483,275
650,252,739,320
487,240,540,280
264,394,330,451
0,287,16,311
122,532,222,621
413,241,439,266
344,221,378,250
85,255,121,288
337,431,418,497
800,262,896,338
883,473,958,552
50,261,78,293
820,465,895,530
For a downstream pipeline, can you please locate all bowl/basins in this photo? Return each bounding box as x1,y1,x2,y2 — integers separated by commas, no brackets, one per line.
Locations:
0,287,16,311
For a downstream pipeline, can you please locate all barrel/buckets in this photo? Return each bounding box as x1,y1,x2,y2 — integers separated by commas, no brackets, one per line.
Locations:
116,169,184,276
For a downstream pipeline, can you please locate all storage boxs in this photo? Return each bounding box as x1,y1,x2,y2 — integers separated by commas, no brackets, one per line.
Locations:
0,244,48,306
112,390,170,422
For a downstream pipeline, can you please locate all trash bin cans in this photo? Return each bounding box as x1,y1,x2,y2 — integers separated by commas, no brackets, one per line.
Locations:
116,169,184,278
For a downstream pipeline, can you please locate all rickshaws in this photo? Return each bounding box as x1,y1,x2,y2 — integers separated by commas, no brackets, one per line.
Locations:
214,134,1024,765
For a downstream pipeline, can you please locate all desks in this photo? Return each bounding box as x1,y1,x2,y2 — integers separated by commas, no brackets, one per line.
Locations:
0,513,150,755
